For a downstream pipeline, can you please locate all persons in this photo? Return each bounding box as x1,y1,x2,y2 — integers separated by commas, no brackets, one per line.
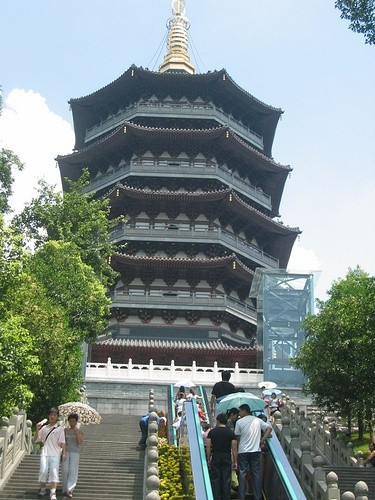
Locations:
33,408,65,500
206,414,237,500
363,442,375,467
138,414,167,447
62,414,83,497
234,404,272,500
258,386,266,399
176,386,211,445
211,372,235,412
269,386,283,422
226,408,239,495
245,414,272,495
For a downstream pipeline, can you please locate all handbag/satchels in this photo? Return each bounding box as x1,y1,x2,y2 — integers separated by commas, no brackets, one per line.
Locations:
40,442,44,448
231,470,239,488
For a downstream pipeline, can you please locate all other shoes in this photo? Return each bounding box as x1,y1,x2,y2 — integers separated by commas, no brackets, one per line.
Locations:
49,488,57,500
39,487,47,496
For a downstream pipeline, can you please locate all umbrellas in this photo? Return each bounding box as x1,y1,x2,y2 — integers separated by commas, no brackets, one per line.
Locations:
262,389,281,395
214,392,267,412
258,381,277,388
